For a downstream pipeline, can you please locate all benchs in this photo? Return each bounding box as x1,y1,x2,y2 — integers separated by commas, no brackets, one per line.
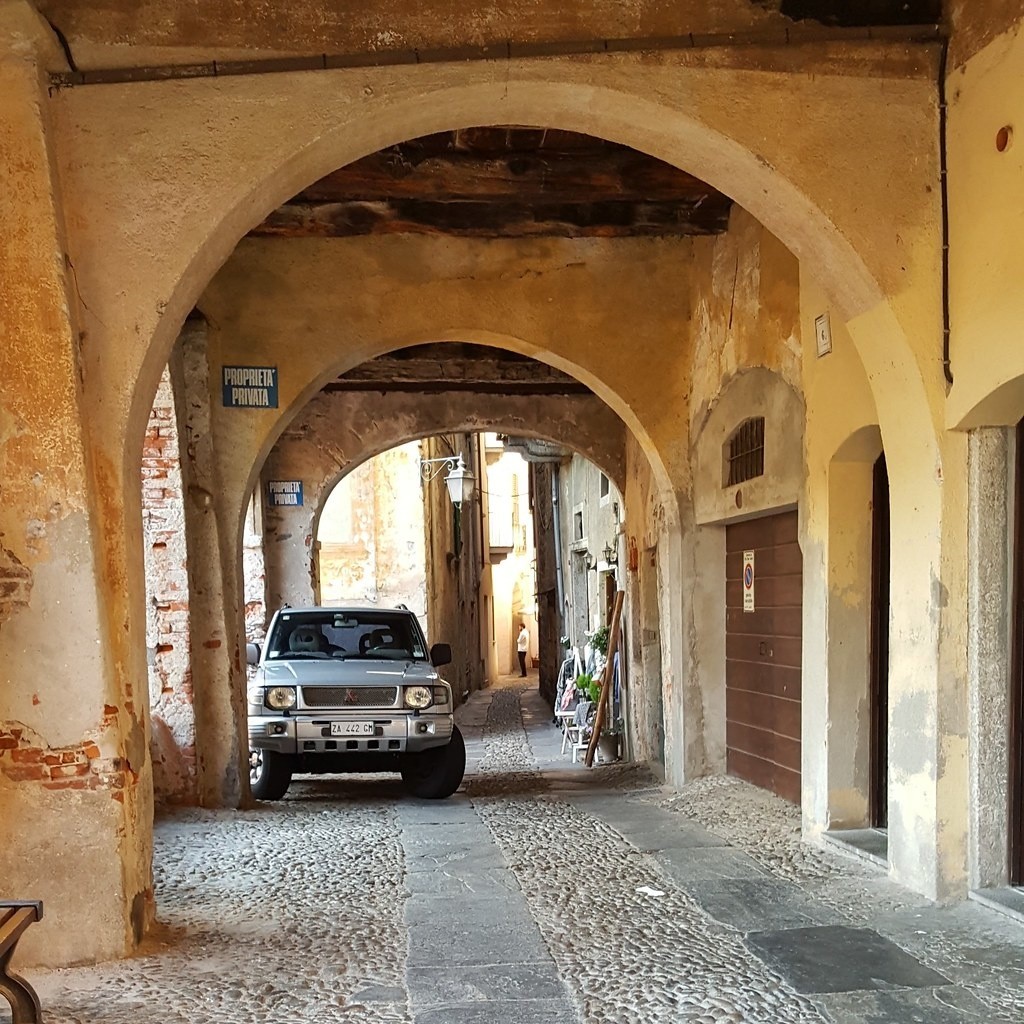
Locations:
320,632,384,659
0,899,45,1024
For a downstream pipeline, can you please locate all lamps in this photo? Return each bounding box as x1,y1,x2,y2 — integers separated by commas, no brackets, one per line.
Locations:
581,549,598,573
602,540,619,568
420,450,477,514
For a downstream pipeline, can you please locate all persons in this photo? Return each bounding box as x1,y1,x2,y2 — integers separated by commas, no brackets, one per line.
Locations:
516,623,529,678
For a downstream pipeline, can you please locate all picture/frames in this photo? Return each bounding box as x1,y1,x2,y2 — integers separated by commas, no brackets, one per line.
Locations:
814,310,832,358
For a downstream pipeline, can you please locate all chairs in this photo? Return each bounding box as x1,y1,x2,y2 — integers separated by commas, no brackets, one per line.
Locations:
569,725,599,763
284,628,329,659
366,629,411,659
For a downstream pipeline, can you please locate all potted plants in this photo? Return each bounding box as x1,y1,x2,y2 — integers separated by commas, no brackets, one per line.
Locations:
577,623,621,766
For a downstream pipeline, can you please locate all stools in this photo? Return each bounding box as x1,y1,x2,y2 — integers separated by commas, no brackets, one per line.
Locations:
555,711,577,756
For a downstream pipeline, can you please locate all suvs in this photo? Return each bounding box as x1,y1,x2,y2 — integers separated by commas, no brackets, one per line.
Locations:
247,603,465,800
